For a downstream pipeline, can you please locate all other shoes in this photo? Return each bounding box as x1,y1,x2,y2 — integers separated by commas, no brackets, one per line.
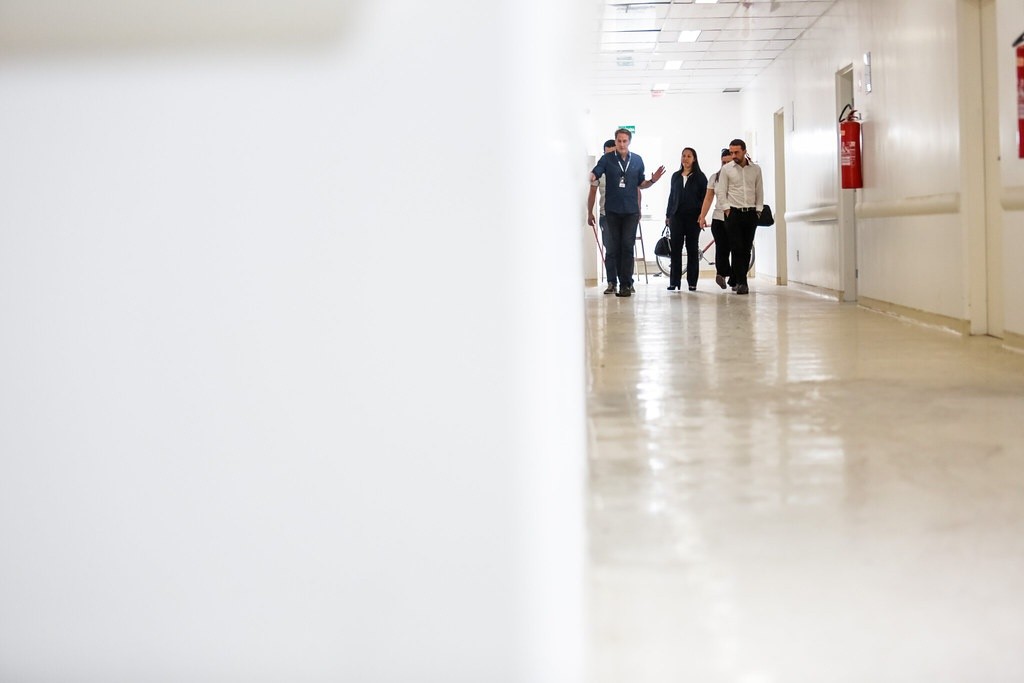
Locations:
627,284,635,293
618,286,631,297
716,275,726,289
737,283,748,294
732,283,738,291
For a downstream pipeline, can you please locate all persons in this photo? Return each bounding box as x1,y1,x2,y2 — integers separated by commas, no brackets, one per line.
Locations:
588,129,667,296
698,139,764,294
665,147,709,290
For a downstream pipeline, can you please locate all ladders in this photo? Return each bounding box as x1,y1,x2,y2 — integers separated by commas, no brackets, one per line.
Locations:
602,221,650,284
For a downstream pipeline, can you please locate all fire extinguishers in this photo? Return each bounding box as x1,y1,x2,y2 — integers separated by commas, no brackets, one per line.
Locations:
1011,32,1024,158
839,104,864,189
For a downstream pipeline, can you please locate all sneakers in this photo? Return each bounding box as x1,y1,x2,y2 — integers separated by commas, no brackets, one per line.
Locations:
604,281,616,293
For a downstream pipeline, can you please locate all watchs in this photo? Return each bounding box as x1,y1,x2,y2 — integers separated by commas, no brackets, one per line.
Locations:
757,211,761,215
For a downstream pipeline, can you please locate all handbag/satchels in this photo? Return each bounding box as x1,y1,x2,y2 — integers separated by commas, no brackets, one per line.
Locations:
654,224,672,258
757,205,774,226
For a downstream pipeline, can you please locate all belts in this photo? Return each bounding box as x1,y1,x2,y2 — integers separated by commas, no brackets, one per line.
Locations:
731,207,756,212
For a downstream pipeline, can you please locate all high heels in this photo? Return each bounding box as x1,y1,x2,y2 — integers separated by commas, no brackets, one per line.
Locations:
667,281,681,290
689,284,696,291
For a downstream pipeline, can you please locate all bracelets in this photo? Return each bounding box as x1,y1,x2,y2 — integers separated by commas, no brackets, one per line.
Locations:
650,179,654,183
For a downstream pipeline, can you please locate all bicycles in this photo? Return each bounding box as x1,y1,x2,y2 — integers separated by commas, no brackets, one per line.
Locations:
655,221,756,278
